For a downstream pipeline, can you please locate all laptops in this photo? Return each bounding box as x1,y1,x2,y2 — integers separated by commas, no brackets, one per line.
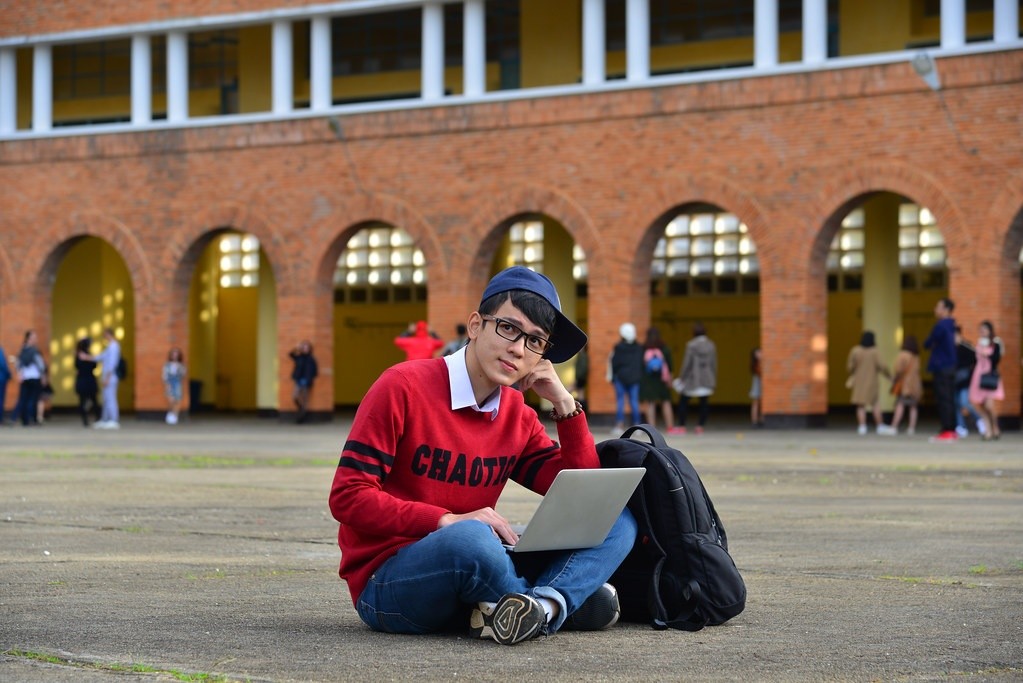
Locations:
502,468,646,553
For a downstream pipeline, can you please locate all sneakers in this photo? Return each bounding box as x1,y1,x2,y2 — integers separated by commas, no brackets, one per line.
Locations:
559,582,621,631
469,593,544,645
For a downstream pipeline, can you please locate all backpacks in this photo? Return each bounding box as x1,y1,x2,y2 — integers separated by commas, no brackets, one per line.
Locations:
595,424,747,632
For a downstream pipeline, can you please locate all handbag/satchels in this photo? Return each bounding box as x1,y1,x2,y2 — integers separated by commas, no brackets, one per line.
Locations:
981,372,999,389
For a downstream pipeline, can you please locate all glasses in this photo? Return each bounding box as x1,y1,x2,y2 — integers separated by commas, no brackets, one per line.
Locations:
480,313,554,356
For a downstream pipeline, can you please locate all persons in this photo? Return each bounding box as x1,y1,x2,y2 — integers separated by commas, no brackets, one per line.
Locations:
748,347,763,428
605,323,718,437
923,298,1005,446
847,331,923,437
288,340,318,424
329,264,638,646
161,346,187,424
75,328,122,430
0,329,54,426
393,320,469,361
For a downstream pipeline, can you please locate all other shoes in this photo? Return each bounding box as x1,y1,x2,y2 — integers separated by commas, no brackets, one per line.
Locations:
83,420,123,430
929,418,1001,442
859,422,916,437
165,411,179,424
611,425,704,437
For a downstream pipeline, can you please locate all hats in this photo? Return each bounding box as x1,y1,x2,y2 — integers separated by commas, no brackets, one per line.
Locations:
619,323,637,345
483,265,589,364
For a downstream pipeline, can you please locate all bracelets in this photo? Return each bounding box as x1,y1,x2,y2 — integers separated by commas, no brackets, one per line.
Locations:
549,400,582,421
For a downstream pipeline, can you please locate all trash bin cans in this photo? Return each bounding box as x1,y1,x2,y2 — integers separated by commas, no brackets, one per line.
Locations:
190,380,202,413
